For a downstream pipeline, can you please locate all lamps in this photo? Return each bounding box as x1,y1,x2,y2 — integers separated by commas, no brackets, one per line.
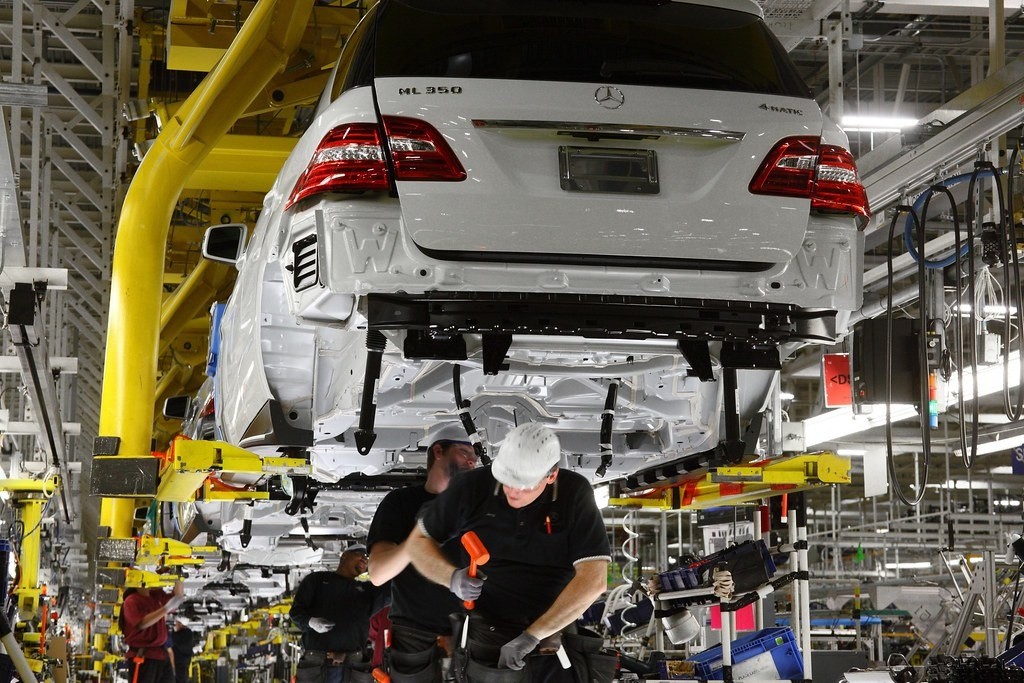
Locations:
822,101,943,133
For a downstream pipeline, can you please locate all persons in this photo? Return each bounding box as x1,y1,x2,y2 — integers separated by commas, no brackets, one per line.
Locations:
290,543,375,683
409,422,613,683
365,425,479,683
117,583,192,683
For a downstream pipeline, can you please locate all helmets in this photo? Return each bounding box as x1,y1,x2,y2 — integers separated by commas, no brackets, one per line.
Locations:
426,423,471,451
343,543,368,553
491,421,560,490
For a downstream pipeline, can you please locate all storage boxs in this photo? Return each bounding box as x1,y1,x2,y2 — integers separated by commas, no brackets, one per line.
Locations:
659,539,776,593
688,627,805,681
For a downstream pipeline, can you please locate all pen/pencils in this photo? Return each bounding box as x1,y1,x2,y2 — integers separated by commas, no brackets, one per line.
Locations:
546,515,552,534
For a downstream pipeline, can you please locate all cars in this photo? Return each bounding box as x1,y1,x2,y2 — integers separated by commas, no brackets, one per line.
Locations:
96,375,388,651
200,0,873,484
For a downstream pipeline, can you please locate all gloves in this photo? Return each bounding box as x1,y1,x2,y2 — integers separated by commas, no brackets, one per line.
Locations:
497,631,540,671
308,617,335,634
165,596,183,611
449,565,487,602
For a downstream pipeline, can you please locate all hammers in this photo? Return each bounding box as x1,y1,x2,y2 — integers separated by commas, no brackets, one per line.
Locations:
132,657,145,683
371,668,390,683
461,531,491,610
